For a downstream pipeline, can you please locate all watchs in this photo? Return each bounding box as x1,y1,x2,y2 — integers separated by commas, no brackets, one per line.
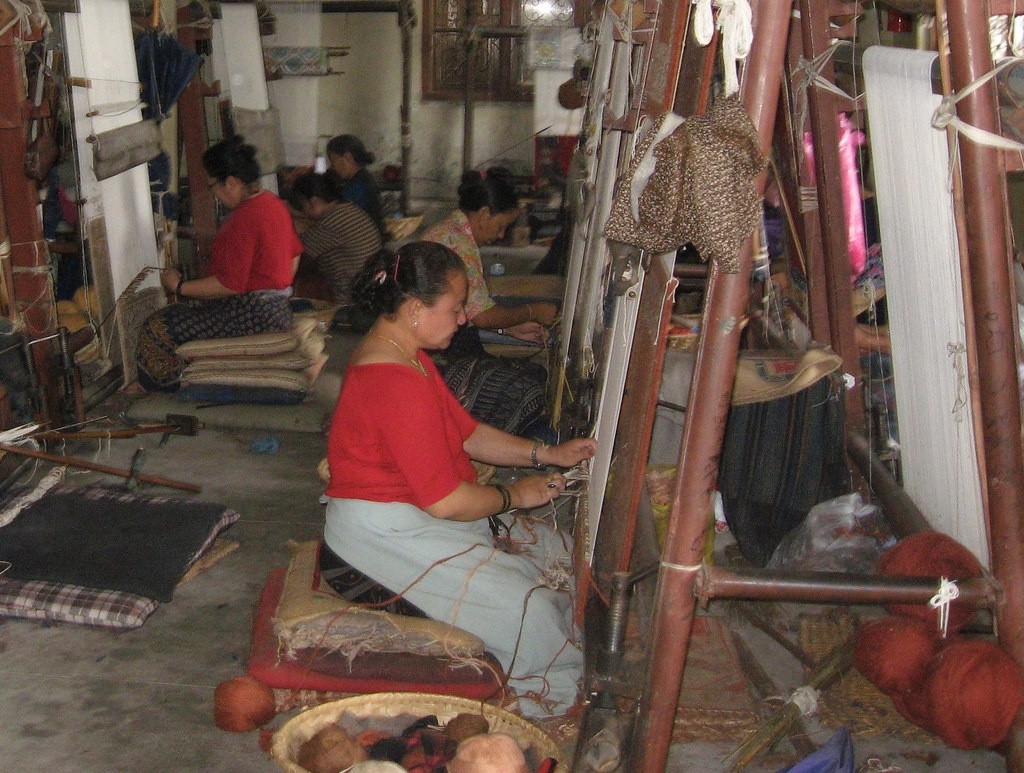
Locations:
532,442,546,471
497,328,503,336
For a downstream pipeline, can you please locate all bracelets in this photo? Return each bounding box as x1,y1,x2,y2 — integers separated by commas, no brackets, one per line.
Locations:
482,483,512,538
176,280,184,296
524,303,533,322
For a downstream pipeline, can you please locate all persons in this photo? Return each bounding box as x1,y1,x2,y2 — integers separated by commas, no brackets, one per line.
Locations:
136,135,304,392
326,133,383,236
324,241,598,717
414,170,557,436
856,323,892,354
290,171,383,307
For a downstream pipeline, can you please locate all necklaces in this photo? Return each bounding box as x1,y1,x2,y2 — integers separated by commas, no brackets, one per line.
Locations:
370,326,427,376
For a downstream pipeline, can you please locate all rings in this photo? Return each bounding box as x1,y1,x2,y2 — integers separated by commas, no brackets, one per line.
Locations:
546,483,559,489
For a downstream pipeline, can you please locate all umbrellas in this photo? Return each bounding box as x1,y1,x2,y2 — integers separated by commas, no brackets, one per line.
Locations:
134,0,206,126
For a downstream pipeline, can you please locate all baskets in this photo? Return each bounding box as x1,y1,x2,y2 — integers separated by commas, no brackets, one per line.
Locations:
271,692,564,773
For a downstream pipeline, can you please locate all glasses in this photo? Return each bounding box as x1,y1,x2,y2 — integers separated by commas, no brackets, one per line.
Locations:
206,176,226,190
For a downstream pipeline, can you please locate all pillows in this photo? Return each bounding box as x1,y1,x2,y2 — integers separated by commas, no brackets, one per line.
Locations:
176,319,333,404
249,538,500,701
